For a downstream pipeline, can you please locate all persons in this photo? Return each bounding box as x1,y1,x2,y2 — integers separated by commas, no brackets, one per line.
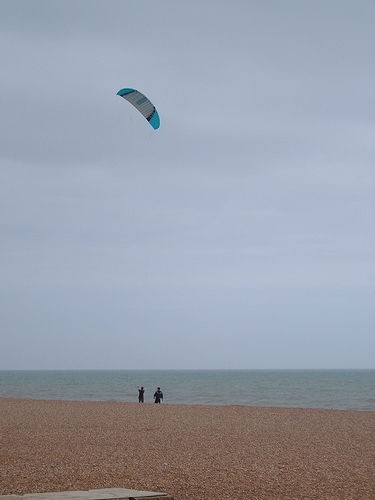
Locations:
154,387,162,403
138,387,144,403
